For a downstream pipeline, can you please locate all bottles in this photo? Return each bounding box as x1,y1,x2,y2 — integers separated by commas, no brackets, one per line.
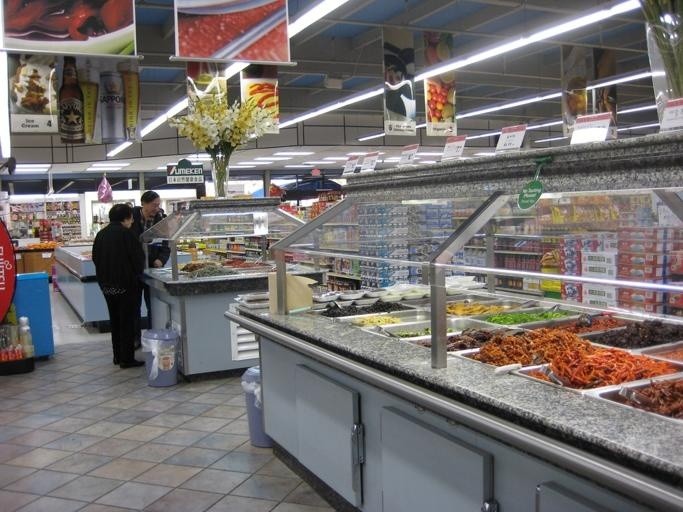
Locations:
56,55,87,145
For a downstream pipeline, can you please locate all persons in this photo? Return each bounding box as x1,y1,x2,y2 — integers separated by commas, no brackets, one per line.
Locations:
125,202,133,208
158,208,167,219
126,190,170,352
90,204,145,369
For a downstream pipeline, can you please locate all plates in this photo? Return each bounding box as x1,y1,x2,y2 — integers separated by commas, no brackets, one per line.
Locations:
233,275,487,311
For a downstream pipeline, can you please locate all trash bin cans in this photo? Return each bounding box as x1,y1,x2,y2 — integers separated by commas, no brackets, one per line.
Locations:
141,330,180,387
241,365,274,447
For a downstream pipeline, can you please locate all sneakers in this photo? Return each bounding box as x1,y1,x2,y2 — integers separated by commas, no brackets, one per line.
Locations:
135,341,140,349
114,359,145,368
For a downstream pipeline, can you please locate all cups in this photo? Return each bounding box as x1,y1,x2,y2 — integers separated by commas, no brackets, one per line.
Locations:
117,60,141,141
76,65,100,146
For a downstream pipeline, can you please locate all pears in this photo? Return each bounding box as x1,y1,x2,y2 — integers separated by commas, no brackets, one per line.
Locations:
436,40,450,61
440,70,454,83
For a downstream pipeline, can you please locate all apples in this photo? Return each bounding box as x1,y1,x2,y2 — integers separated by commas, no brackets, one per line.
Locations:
427,47,438,65
427,79,456,124
